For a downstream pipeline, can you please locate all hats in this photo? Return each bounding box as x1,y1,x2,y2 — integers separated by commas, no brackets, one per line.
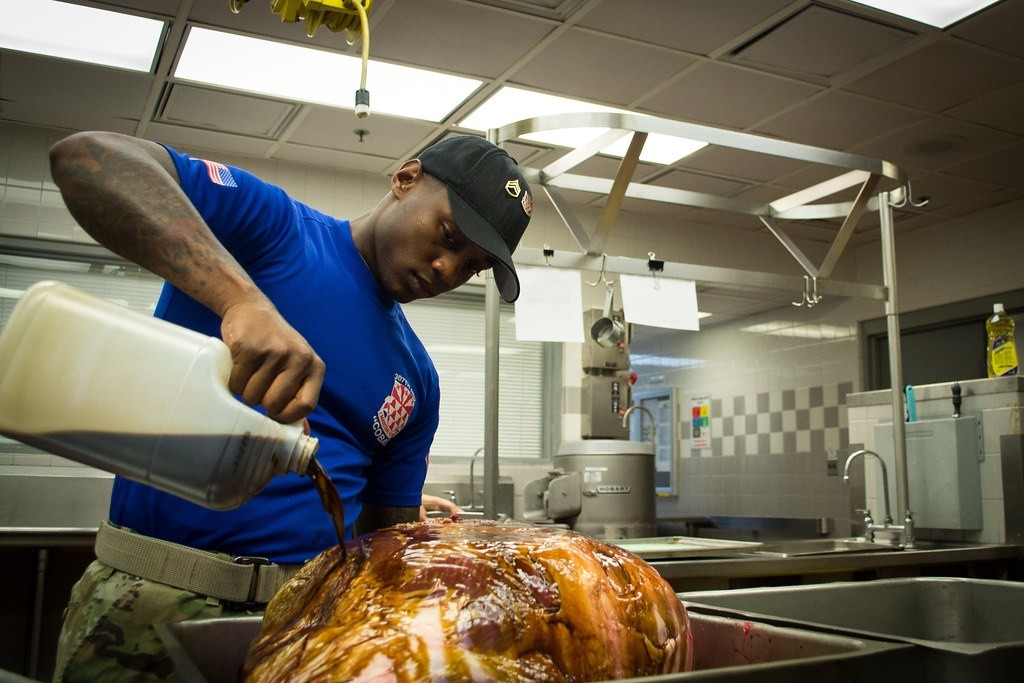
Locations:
416,135,533,304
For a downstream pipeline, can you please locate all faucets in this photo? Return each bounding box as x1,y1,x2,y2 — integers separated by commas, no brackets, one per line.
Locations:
622,404,655,442
843,448,894,527
469,446,486,509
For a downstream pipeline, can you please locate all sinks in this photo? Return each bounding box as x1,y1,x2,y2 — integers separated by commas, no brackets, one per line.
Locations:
737,538,904,559
679,576,1024,663
158,601,873,681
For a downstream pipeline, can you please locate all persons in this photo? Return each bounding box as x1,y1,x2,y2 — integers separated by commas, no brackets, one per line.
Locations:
47,130,533,683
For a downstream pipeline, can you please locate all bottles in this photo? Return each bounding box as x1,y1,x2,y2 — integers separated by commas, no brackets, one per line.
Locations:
0,280,319,510
986,303,1019,379
